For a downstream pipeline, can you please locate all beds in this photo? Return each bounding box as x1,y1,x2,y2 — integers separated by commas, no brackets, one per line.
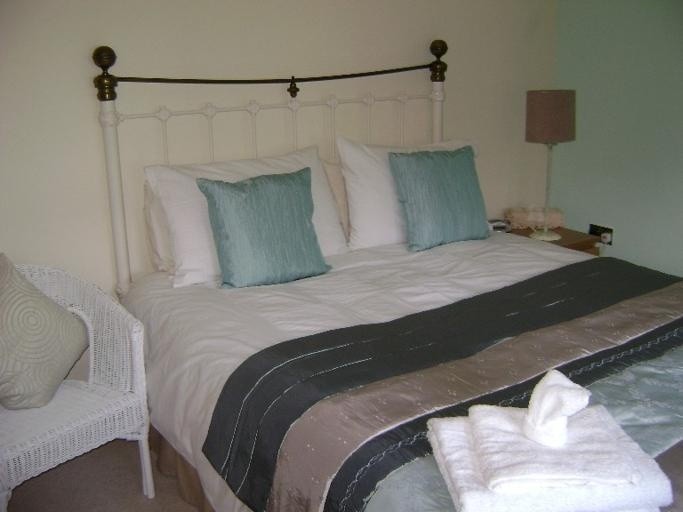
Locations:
91,38,681,512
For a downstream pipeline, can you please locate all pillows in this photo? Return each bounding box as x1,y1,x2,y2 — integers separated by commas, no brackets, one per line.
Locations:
0,255,89,411
332,134,480,250
144,146,346,287
196,167,331,289
386,144,488,254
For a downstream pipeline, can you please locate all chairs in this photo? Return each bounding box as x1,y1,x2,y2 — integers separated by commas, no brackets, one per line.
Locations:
0,264,155,512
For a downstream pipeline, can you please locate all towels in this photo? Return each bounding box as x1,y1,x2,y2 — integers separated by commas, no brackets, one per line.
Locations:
426,416,665,512
521,369,588,441
464,401,671,510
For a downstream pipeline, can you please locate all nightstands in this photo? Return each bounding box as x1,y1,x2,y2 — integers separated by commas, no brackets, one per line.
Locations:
489,221,602,256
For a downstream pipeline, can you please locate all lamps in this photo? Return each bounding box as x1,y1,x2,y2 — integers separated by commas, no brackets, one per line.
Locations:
523,88,575,241
